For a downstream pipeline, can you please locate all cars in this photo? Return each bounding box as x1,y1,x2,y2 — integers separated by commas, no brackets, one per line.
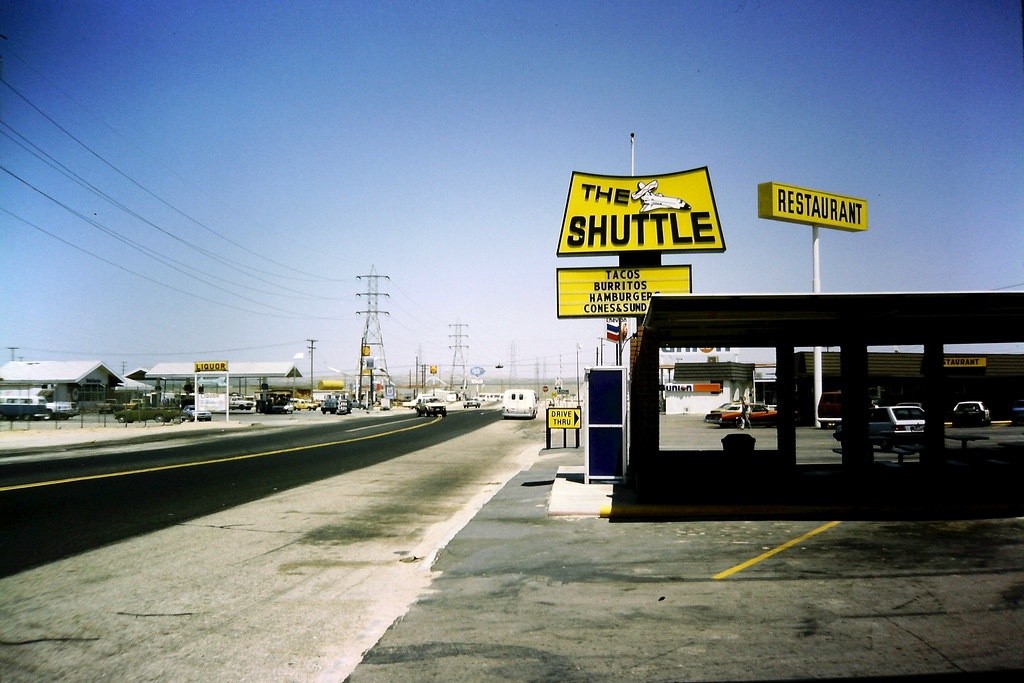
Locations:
181,406,213,422
462,398,481,408
832,405,926,449
1010,400,1024,424
272,400,293,414
952,401,991,427
896,403,923,415
704,401,802,429
322,399,339,414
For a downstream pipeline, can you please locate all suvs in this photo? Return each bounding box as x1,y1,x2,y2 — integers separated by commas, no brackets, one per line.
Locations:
817,391,845,427
415,397,447,417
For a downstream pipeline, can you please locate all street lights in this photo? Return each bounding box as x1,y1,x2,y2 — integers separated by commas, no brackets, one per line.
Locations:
576,341,583,407
328,367,359,401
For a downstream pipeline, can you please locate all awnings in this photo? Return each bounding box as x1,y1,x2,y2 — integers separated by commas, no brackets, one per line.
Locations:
37,390,54,397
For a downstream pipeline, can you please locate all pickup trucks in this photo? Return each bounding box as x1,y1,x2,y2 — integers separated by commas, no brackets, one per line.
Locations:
113,404,181,424
43,401,79,421
122,400,146,409
350,399,366,409
230,396,254,410
290,399,319,411
94,399,124,414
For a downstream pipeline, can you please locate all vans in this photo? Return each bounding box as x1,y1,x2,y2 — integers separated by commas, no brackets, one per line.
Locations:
0,395,48,420
502,389,539,419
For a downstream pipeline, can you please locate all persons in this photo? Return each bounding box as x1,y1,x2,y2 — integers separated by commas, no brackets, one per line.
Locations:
735,396,752,430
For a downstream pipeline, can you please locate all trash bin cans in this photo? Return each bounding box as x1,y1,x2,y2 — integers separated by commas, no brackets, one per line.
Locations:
721,434,756,451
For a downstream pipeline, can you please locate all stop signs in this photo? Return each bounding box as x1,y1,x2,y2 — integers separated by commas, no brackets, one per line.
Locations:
542,386,548,392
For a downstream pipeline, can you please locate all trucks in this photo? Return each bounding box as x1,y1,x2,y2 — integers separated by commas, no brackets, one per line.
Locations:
403,394,434,409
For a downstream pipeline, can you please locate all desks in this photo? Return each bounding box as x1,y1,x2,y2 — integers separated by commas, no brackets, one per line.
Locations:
945,435,990,448
832,432,924,464
